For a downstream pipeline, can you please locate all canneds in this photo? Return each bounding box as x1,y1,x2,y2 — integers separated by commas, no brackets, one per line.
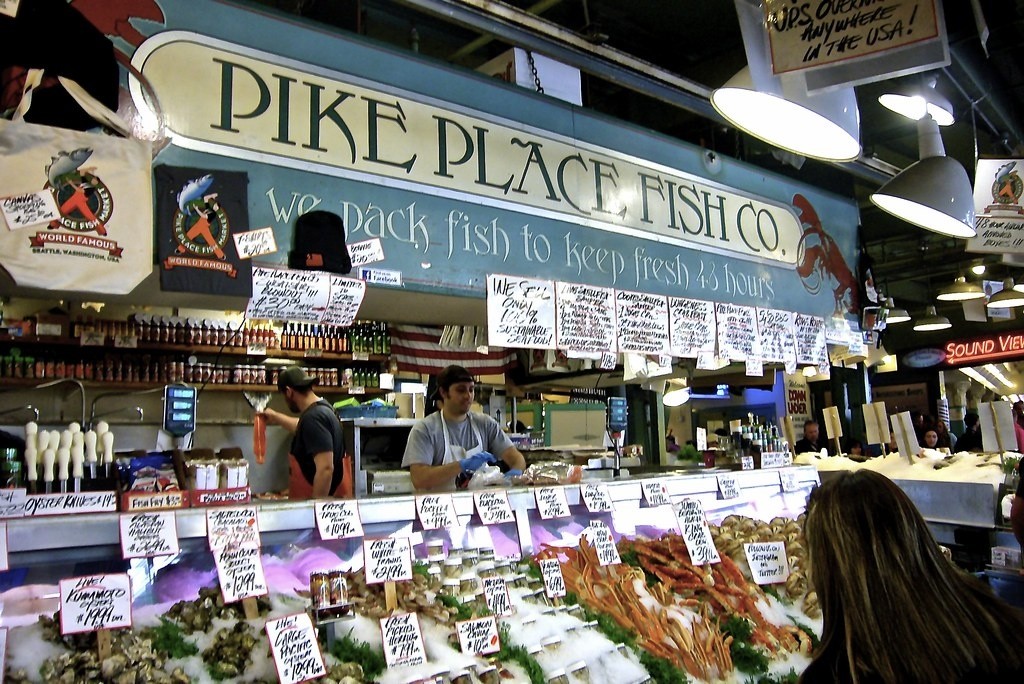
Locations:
310,567,349,618
401,538,657,684
0,318,338,386
741,415,788,452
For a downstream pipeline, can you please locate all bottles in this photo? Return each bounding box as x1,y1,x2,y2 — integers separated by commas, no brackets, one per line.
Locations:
1012,463,1019,493
73,315,391,387
741,412,790,453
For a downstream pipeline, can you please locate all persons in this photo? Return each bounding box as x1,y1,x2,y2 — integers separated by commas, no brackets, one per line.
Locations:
1001,457,1024,584
509,421,527,433
1012,401,1024,453
910,412,983,452
666,435,693,452
796,469,1024,684
795,421,831,456
255,365,353,499
400,365,526,492
842,425,882,457
887,428,898,454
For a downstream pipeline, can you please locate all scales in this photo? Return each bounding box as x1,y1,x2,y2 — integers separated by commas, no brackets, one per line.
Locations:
581,397,630,478
163,386,197,451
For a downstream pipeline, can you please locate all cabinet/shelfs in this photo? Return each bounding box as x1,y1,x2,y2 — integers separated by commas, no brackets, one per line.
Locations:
0,329,401,393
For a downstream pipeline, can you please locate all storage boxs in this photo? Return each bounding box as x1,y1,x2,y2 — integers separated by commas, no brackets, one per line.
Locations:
117,447,251,509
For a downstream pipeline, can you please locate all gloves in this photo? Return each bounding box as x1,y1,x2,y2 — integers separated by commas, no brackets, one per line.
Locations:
504,470,522,479
459,450,496,471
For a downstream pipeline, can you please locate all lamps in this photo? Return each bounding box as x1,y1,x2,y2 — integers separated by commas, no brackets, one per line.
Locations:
868,117,975,239
986,272,1024,308
660,378,695,407
714,63,863,163
881,242,911,323
802,365,816,377
878,71,958,127
936,239,983,302
913,240,952,332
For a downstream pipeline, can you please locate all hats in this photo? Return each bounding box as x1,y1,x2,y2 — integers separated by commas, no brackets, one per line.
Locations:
430,365,475,401
279,366,320,387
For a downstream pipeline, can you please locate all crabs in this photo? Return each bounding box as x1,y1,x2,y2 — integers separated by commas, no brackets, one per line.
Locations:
532,511,821,679
